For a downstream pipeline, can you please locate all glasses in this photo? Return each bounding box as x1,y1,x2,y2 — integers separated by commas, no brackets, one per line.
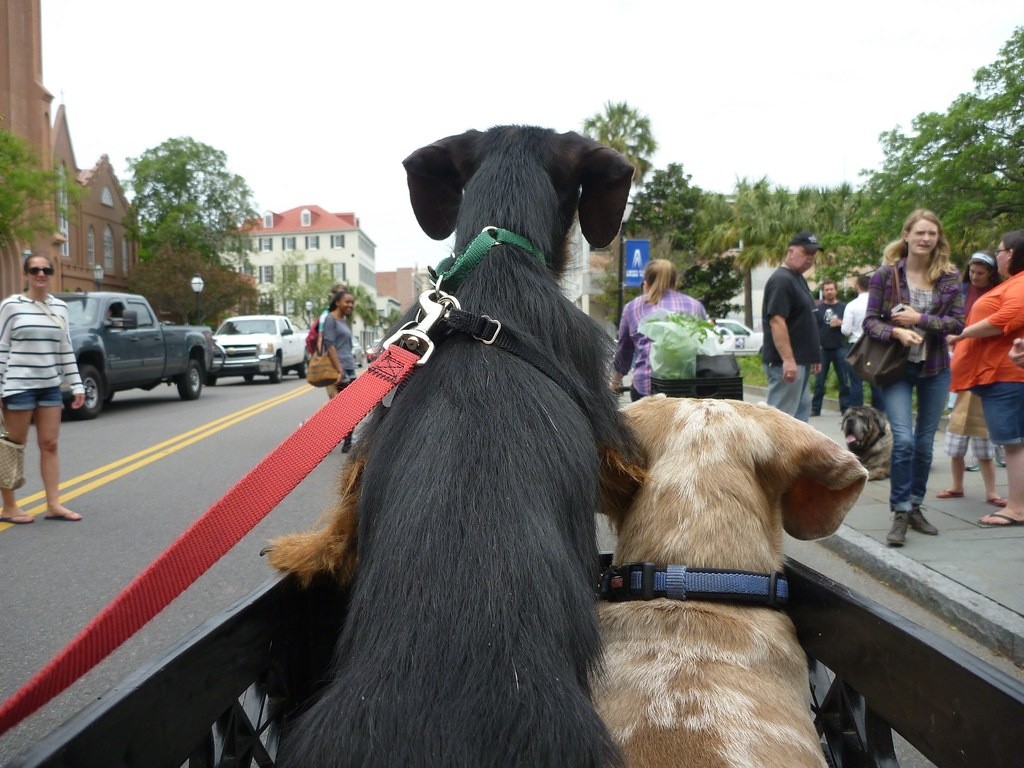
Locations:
26,266,54,276
993,248,1009,255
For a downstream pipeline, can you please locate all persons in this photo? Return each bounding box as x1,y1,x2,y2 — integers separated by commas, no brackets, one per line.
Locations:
809,280,851,418
322,291,362,453
945,228,1024,526
937,389,1009,509
840,275,885,418
947,251,1003,352
759,235,824,422
318,284,349,400
611,259,713,401
0,254,86,525
862,207,967,543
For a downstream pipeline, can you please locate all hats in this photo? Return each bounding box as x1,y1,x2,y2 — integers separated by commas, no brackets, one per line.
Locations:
787,233,826,254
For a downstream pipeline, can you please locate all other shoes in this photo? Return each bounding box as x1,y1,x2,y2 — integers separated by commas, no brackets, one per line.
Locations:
810,410,820,416
887,511,907,545
905,505,938,536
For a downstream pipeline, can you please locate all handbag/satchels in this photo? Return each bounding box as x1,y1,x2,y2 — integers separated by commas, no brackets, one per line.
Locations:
845,321,911,388
0,433,24,490
637,310,740,380
307,351,339,387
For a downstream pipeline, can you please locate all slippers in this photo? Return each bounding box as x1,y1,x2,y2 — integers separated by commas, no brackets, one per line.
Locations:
45,507,81,520
976,510,1024,526
935,490,963,498
1,507,32,525
986,496,1007,507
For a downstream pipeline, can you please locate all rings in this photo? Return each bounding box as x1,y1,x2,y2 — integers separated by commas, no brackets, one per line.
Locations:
787,374,791,377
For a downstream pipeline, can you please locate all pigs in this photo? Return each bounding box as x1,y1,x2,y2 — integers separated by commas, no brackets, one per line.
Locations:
263,125,632,768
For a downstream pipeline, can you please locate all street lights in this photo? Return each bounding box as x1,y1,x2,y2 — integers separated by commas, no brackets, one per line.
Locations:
191,272,204,325
94,264,103,291
616,195,634,340
305,300,313,330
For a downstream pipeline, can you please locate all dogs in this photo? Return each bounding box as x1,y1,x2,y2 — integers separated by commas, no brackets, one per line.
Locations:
840,406,893,481
586,391,867,768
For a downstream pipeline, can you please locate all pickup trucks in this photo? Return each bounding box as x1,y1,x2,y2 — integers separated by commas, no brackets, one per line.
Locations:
204,315,312,386
50,292,214,420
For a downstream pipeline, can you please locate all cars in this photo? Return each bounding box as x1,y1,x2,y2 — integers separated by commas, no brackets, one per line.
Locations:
352,337,382,368
715,319,764,356
